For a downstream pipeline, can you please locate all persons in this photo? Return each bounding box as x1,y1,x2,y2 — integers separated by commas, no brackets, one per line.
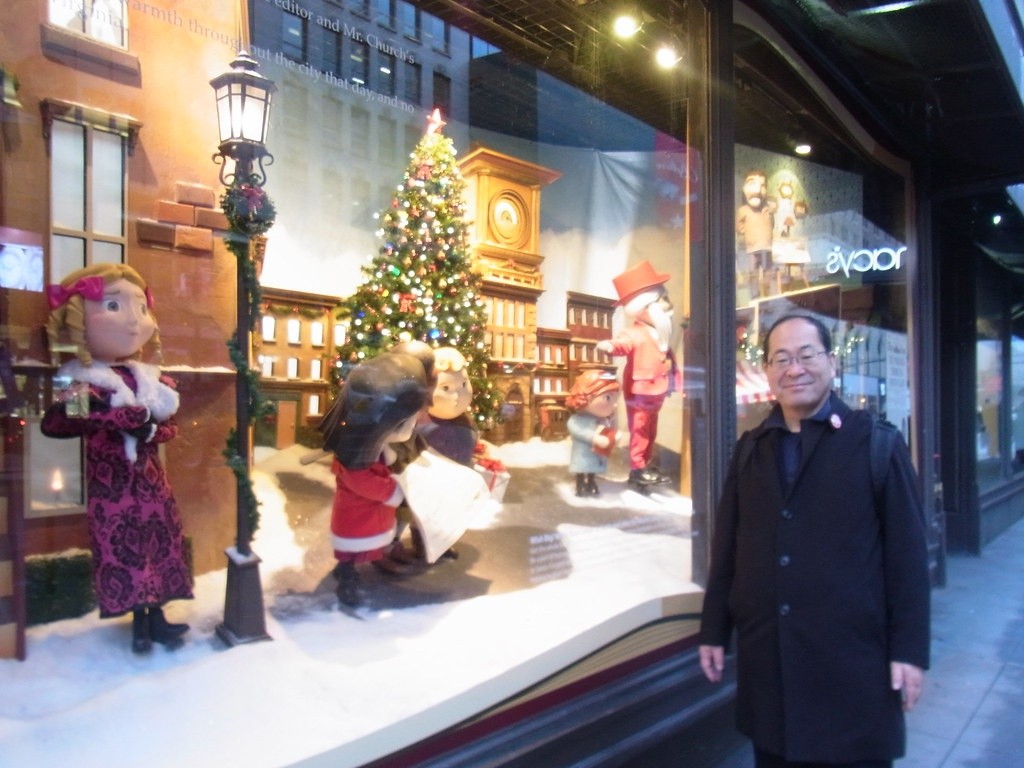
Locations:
696,315,931,768
566,369,623,499
315,350,432,608
40,262,195,653
374,340,443,568
411,344,484,563
736,168,777,303
595,260,682,487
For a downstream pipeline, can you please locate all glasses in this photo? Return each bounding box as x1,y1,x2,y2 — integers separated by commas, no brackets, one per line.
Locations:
767,350,825,368
